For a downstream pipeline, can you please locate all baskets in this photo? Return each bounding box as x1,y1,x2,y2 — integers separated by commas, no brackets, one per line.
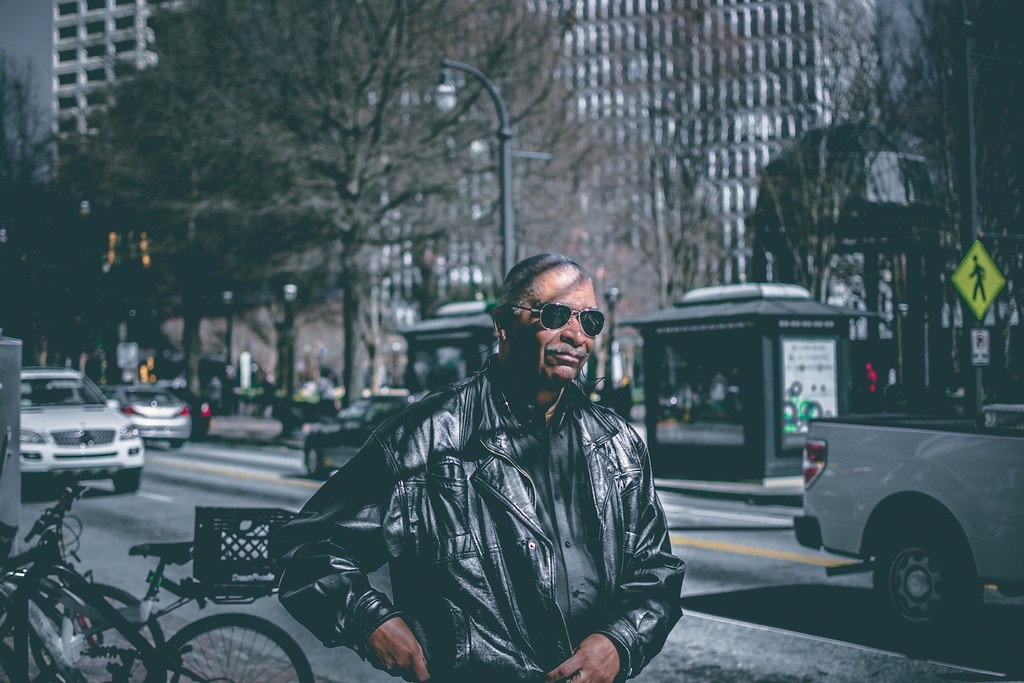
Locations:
193,506,298,584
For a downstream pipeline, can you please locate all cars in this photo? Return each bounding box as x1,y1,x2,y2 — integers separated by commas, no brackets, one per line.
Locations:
21,365,213,494
302,390,421,478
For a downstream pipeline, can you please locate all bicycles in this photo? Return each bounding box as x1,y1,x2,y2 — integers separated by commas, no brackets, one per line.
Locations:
0,484,316,683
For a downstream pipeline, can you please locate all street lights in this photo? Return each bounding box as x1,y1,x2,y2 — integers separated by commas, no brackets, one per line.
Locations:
222,290,233,417
606,283,623,409
279,284,297,439
433,59,516,281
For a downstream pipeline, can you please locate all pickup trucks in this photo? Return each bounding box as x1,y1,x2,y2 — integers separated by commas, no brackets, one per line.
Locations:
793,404,1024,634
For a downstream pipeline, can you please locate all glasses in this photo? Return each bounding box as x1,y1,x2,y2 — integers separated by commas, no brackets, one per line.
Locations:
502,303,606,338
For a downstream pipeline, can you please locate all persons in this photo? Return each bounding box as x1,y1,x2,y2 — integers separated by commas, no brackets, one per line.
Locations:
865,362,877,392
274,251,685,683
